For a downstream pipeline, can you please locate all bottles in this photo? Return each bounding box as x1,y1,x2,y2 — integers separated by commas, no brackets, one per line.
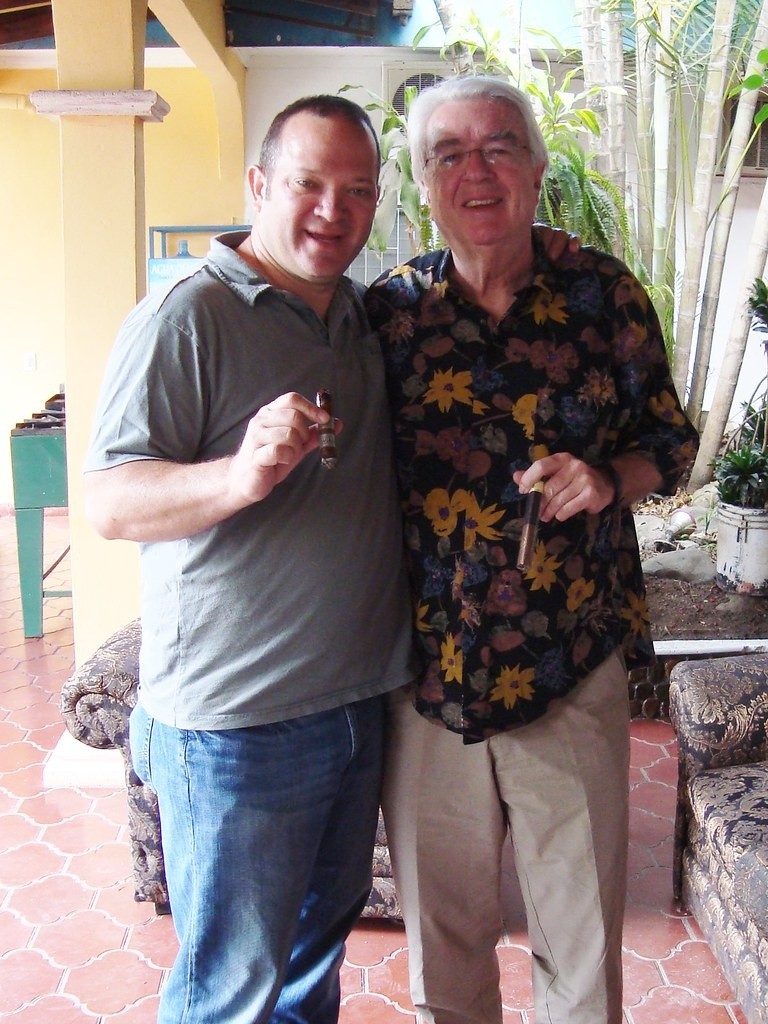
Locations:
174,239,194,257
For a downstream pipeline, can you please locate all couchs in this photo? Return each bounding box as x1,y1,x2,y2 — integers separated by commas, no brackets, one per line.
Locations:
60,616,404,921
668,651,768,1024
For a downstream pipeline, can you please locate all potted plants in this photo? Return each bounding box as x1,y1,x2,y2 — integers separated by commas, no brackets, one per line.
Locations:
707,445,768,598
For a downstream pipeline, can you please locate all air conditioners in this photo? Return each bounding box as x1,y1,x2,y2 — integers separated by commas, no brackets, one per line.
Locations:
380,61,481,134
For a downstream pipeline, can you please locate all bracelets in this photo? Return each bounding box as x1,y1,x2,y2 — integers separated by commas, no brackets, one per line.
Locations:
589,459,626,520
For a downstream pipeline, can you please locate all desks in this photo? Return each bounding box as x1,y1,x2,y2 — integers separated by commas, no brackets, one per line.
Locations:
10,425,67,638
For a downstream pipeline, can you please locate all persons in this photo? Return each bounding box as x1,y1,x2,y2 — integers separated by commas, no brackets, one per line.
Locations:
374,74,698,1024
83,96,409,1024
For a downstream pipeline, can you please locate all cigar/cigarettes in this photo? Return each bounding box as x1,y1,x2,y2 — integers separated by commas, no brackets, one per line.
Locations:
314,383,339,469
517,475,548,575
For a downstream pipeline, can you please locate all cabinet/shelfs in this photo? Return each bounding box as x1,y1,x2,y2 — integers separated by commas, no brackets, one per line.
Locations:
147,223,253,292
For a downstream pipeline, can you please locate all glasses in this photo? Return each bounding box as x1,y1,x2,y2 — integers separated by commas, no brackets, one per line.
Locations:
422,135,537,174
